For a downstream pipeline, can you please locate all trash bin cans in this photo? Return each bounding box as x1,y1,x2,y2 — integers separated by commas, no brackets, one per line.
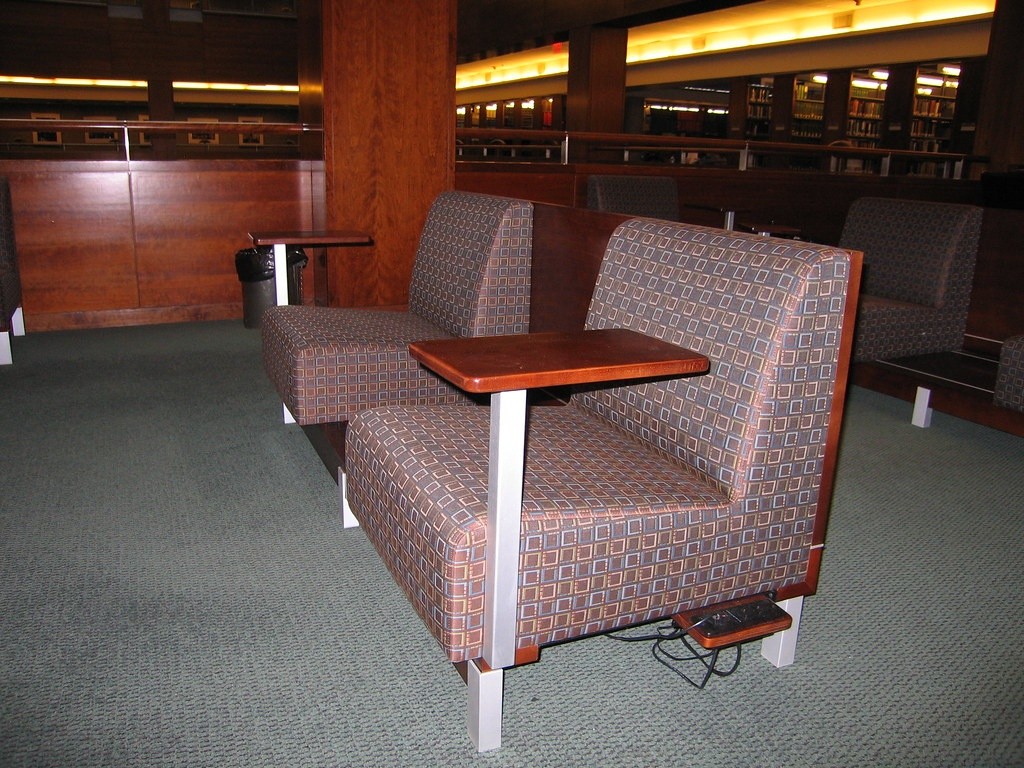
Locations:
238,247,304,330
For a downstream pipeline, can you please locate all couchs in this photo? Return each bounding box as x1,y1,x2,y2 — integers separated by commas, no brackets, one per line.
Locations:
581,174,681,222
830,192,984,366
344,215,867,756
258,187,536,479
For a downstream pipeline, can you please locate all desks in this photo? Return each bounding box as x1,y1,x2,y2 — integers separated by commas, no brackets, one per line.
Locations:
245,231,370,305
408,327,711,672
736,220,801,237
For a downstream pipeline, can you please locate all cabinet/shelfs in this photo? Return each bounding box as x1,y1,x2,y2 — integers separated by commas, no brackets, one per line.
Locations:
626,53,965,173
